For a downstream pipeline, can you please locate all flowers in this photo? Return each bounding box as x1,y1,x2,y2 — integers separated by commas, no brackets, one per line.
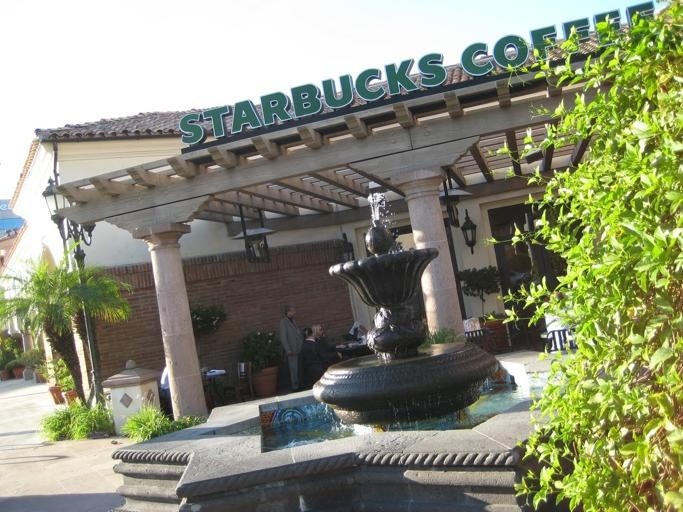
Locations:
242,329,283,369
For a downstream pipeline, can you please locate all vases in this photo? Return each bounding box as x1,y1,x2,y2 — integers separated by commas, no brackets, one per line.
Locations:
250,366,280,398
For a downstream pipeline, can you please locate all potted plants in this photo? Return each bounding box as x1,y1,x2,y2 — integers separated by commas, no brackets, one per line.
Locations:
453,265,511,353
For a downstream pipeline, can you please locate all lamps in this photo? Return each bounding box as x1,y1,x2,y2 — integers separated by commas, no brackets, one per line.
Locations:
460,208,477,255
339,233,355,262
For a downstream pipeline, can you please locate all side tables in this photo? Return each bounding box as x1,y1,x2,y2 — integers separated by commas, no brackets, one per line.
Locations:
335,341,372,359
201,369,225,409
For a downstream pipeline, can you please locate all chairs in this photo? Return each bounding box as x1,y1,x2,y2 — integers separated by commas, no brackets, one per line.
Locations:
222,360,254,404
160,388,175,420
461,317,487,352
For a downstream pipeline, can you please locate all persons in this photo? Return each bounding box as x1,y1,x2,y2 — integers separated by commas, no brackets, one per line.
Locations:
279,305,347,391
